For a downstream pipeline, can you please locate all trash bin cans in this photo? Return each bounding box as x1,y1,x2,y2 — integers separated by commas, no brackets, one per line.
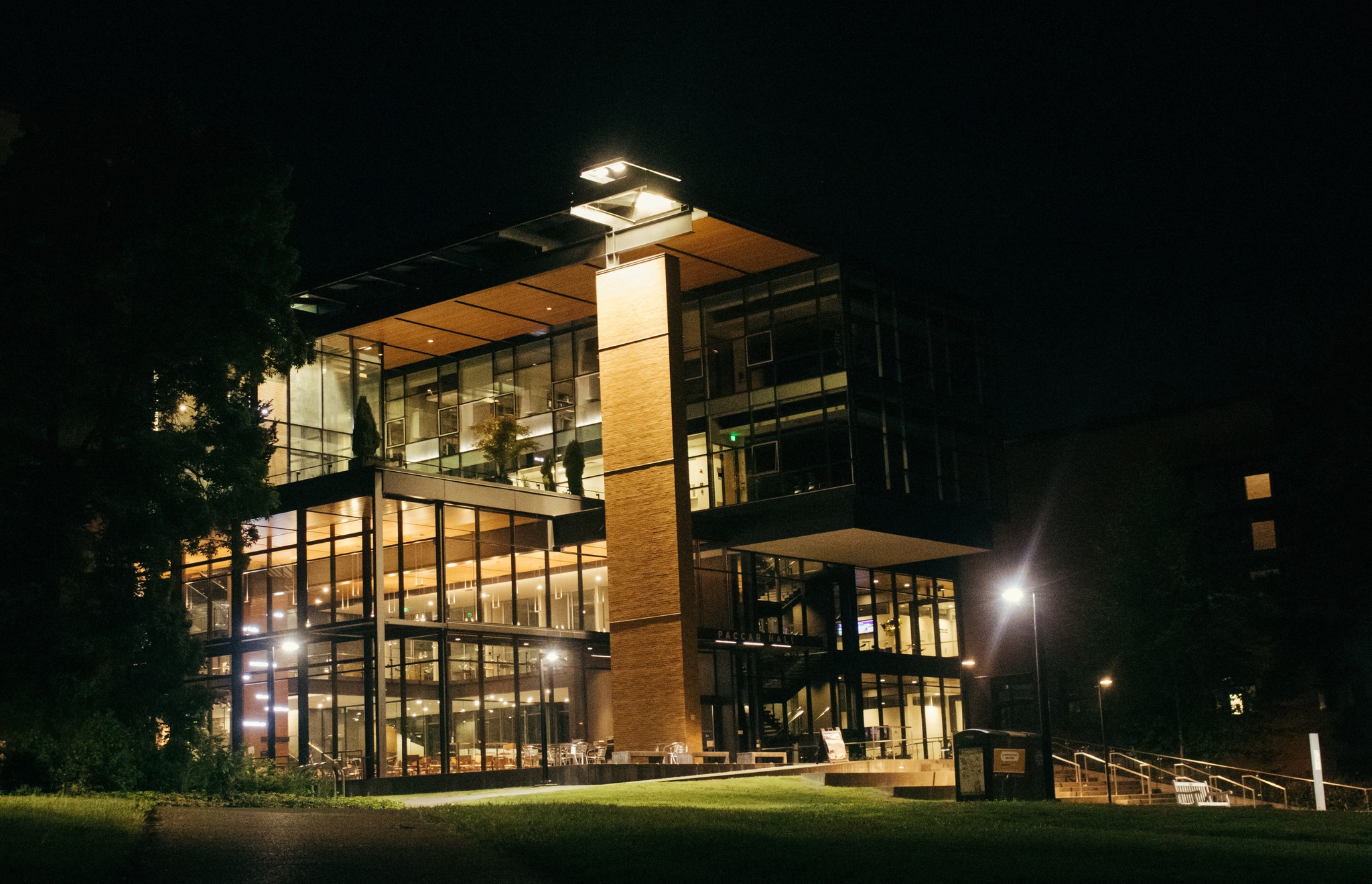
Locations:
1021,732,1046,801
953,729,1010,802
999,729,1026,802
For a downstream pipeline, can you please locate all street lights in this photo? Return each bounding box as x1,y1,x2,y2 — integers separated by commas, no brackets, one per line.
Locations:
961,659,975,730
1002,586,1056,802
1098,679,1114,803
243,626,298,777
539,652,559,784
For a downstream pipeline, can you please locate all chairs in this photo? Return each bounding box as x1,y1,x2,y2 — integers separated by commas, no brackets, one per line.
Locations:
663,742,688,764
322,739,607,781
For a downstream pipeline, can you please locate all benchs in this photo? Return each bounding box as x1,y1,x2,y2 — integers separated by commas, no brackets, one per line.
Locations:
737,752,787,764
612,751,668,764
677,752,729,764
1171,780,1233,807
